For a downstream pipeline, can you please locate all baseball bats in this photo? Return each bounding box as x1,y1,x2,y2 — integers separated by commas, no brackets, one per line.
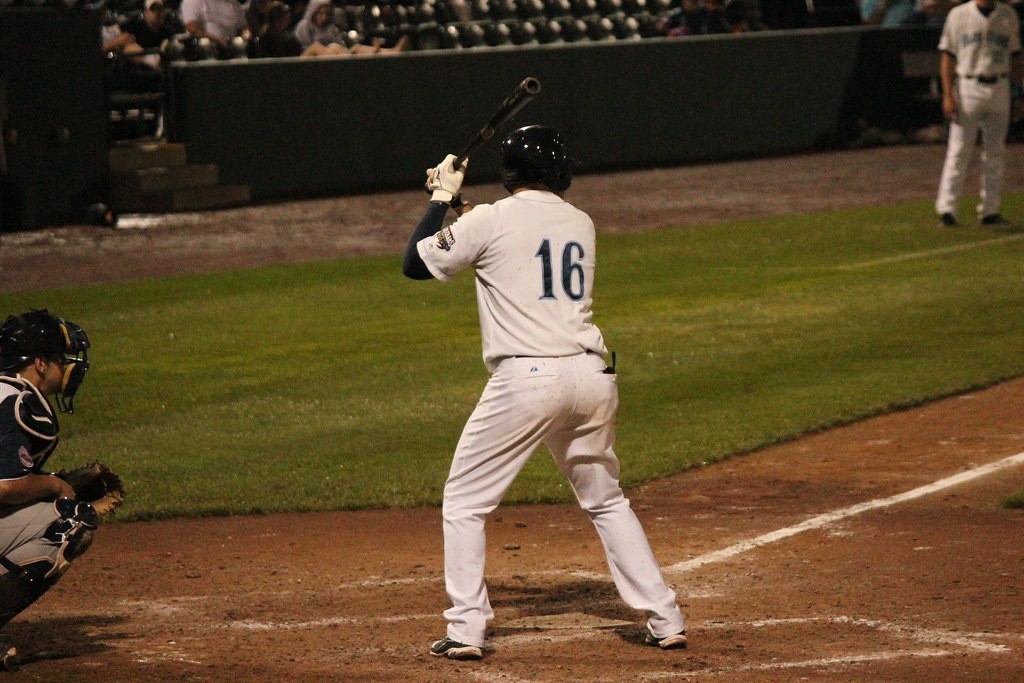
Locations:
453,77,541,169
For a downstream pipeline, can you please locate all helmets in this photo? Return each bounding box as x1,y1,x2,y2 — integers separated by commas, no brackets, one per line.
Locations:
0,307,90,414
500,125,575,190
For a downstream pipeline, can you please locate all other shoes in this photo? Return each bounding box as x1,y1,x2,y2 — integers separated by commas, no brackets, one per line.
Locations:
430,636,483,658
644,629,687,649
940,213,956,226
982,215,1000,223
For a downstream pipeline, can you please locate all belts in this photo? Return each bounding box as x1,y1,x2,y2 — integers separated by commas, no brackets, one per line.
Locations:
966,74,1005,83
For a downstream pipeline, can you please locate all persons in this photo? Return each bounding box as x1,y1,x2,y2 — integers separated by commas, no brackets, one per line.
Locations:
97,0,1024,61
934,0,1024,230
403,126,689,660
0,309,125,671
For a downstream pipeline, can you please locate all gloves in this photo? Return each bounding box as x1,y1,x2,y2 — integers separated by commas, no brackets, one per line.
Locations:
429,153,468,205
426,168,436,191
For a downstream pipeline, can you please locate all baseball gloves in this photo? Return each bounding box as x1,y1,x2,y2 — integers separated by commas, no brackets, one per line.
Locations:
56,456,126,515
